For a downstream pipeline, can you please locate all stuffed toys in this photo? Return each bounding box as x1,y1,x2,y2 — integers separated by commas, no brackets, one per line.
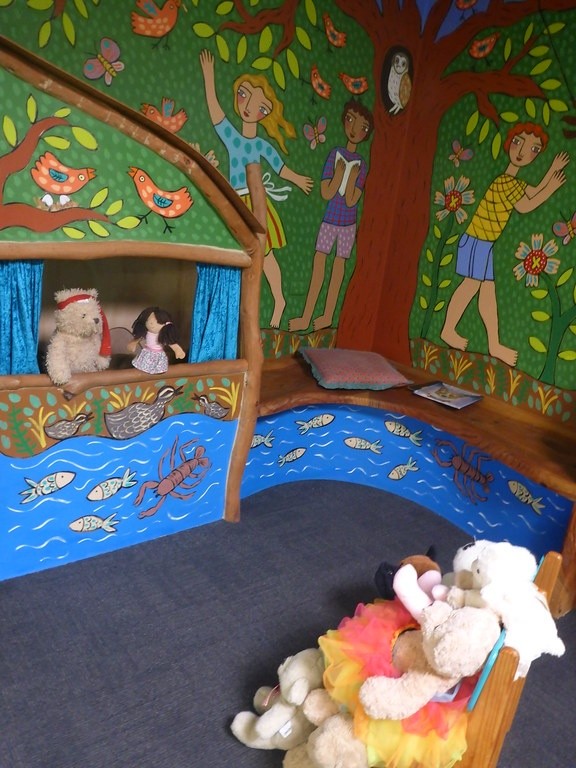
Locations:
230,539,567,768
45,285,113,383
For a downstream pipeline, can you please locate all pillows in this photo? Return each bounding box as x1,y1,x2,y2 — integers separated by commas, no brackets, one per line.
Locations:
298,346,415,390
